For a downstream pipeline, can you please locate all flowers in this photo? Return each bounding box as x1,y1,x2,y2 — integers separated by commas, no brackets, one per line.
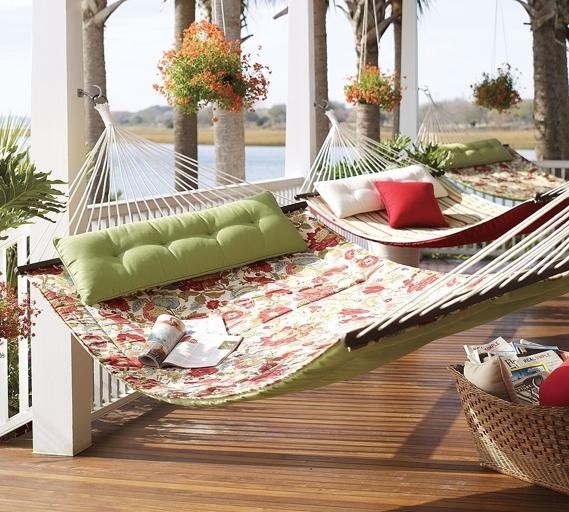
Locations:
153,20,274,125
468,58,527,122
342,61,408,113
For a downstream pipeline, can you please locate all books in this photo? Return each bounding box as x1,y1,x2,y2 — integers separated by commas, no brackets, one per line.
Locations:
462,326,568,407
138,313,244,368
179,316,229,336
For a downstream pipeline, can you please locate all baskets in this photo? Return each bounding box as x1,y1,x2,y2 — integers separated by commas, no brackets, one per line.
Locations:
446,365,569,495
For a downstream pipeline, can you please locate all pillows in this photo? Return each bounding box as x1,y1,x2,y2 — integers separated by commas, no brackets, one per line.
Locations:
310,137,514,229
51,187,307,307
